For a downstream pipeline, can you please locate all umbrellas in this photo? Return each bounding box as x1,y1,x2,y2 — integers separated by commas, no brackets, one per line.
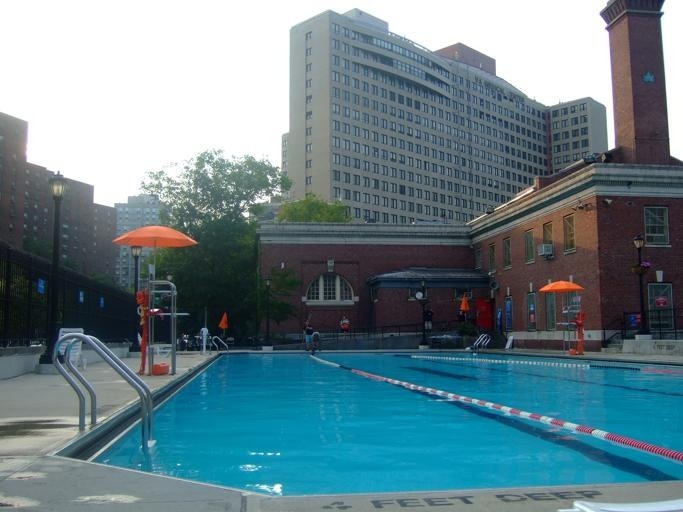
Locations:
538,278,583,316
218,310,229,335
459,293,469,318
111,225,199,279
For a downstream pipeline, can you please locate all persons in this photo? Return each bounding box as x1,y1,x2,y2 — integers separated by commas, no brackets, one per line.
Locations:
304,323,314,353
423,304,433,333
339,315,350,333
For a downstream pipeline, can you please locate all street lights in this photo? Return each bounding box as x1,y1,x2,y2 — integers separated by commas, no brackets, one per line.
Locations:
264,278,272,346
129,246,142,352
633,234,651,335
420,280,430,345
39,171,66,363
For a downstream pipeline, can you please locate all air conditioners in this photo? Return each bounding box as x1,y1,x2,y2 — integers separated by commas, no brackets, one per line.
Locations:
536,243,553,257
